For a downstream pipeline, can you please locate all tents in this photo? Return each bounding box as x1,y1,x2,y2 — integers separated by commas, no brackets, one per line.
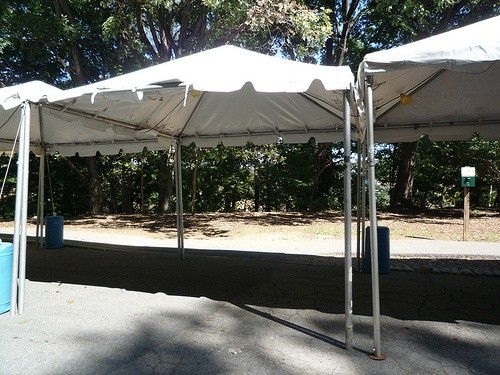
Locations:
18,44,361,352
356,13,500,360
0,80,180,316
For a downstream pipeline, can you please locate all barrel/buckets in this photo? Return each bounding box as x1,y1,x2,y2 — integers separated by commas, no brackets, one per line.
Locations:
0,242,14,314
45,216,64,249
363,226,390,275
